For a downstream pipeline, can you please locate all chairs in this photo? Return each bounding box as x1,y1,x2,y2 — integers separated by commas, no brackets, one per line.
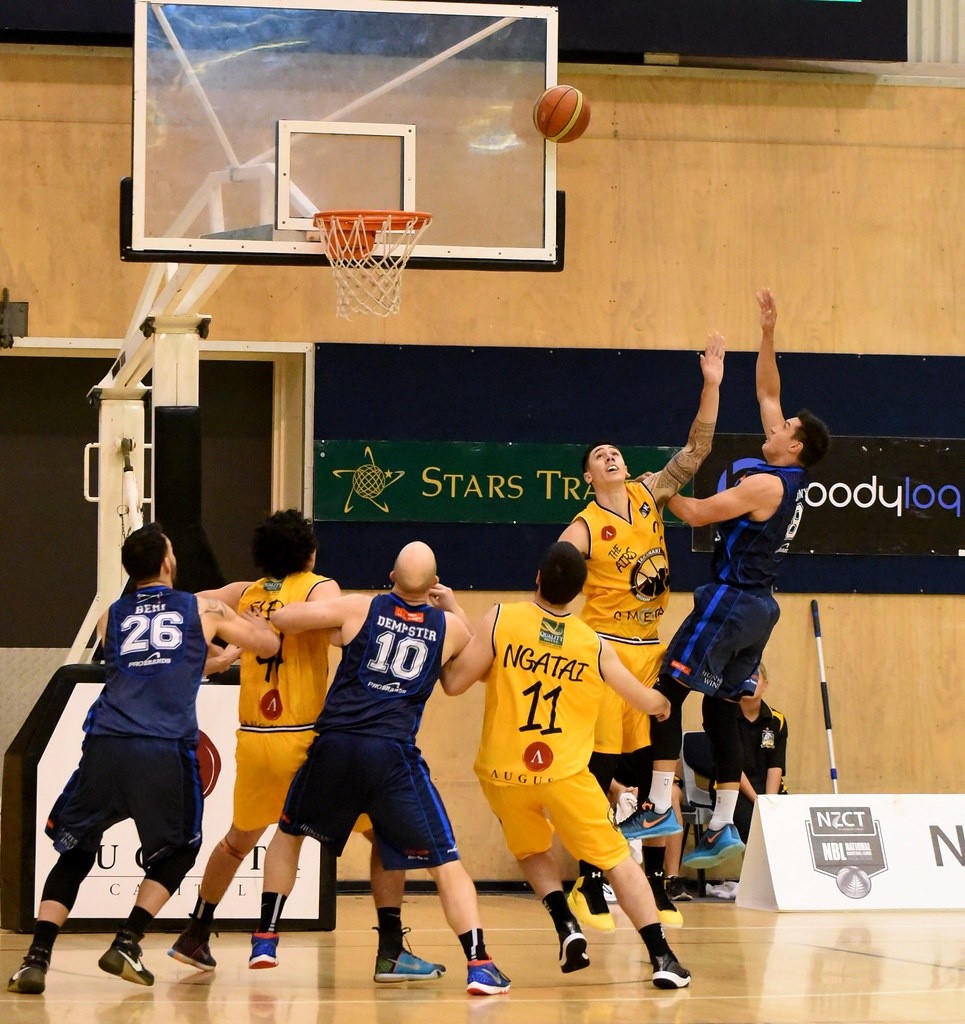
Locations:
682,731,715,898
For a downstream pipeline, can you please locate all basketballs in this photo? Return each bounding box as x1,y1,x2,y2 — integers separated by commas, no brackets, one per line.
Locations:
532,84,592,145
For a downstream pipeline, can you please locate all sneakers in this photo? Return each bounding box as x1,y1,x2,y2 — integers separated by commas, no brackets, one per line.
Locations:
564,874,615,935
558,919,591,975
652,952,693,989
167,971,216,1003
681,824,746,869
8,955,48,996
651,895,684,929
248,930,280,970
466,994,511,1013
650,989,690,1009
372,986,441,1009
93,993,153,1024
615,798,684,841
663,876,694,901
166,934,217,971
601,881,618,902
374,949,447,983
465,956,511,995
97,943,156,987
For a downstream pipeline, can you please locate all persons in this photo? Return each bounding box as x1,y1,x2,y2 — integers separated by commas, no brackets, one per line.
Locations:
250,542,510,995
559,331,725,933
440,541,692,989
7,522,280,993
165,508,447,983
608,776,692,901
708,661,790,845
616,285,829,869
